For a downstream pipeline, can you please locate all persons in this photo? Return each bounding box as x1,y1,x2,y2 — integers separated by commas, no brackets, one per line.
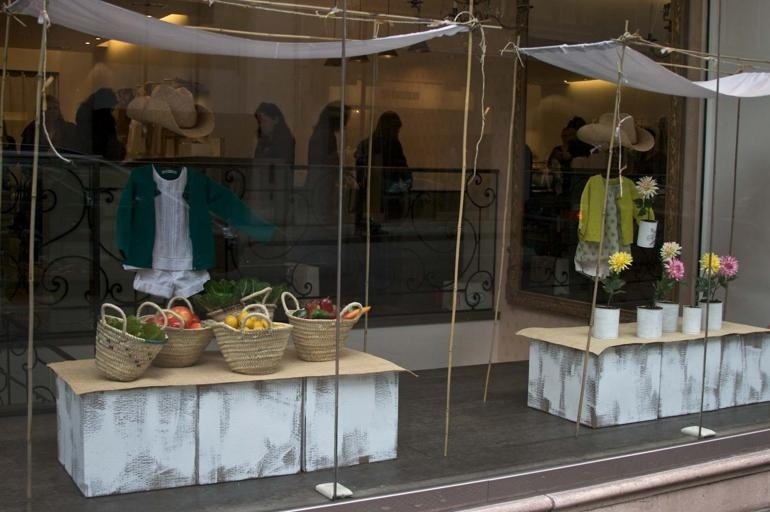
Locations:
304,99,353,271
21,95,86,155
542,127,578,169
351,111,413,310
548,115,598,185
75,88,127,164
249,101,295,287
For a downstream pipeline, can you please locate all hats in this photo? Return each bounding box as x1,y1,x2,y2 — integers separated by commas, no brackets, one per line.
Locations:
126,83,216,138
576,112,655,153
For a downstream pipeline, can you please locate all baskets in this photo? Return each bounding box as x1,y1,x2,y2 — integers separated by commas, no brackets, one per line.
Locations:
206,286,278,321
95,301,168,382
281,291,363,362
213,304,294,375
139,296,212,368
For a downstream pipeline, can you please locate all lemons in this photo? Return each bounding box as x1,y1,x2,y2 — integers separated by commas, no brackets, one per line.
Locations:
222,304,271,331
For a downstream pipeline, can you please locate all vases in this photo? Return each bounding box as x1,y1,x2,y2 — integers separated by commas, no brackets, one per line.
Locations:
637,219,659,249
636,298,723,340
591,306,620,340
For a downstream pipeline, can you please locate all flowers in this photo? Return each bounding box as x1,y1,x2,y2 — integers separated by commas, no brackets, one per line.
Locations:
646,240,740,309
599,251,633,308
632,175,660,221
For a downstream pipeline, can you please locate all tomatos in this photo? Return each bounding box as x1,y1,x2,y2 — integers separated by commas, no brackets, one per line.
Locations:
154,300,203,331
